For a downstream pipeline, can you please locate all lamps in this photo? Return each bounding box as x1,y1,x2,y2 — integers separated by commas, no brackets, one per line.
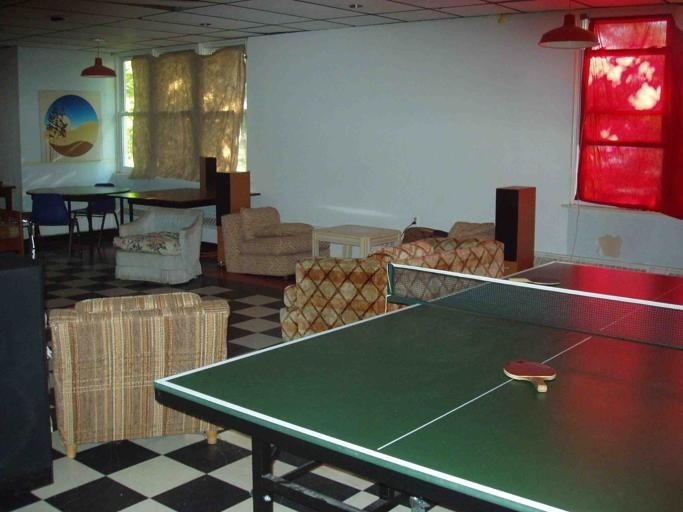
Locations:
80,37,118,79
537,0,600,49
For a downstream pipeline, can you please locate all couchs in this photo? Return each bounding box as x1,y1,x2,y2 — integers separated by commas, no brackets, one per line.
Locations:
280,237,504,342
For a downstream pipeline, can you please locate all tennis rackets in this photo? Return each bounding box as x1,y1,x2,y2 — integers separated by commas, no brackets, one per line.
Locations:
508,277,559,286
503,360,557,393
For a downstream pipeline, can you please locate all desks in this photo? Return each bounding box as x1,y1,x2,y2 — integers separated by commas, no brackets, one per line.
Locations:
107,189,261,224
25,186,130,255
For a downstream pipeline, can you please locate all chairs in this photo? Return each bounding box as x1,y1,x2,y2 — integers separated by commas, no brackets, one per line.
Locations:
31,182,120,260
113,206,203,284
49,291,228,456
220,207,495,280
